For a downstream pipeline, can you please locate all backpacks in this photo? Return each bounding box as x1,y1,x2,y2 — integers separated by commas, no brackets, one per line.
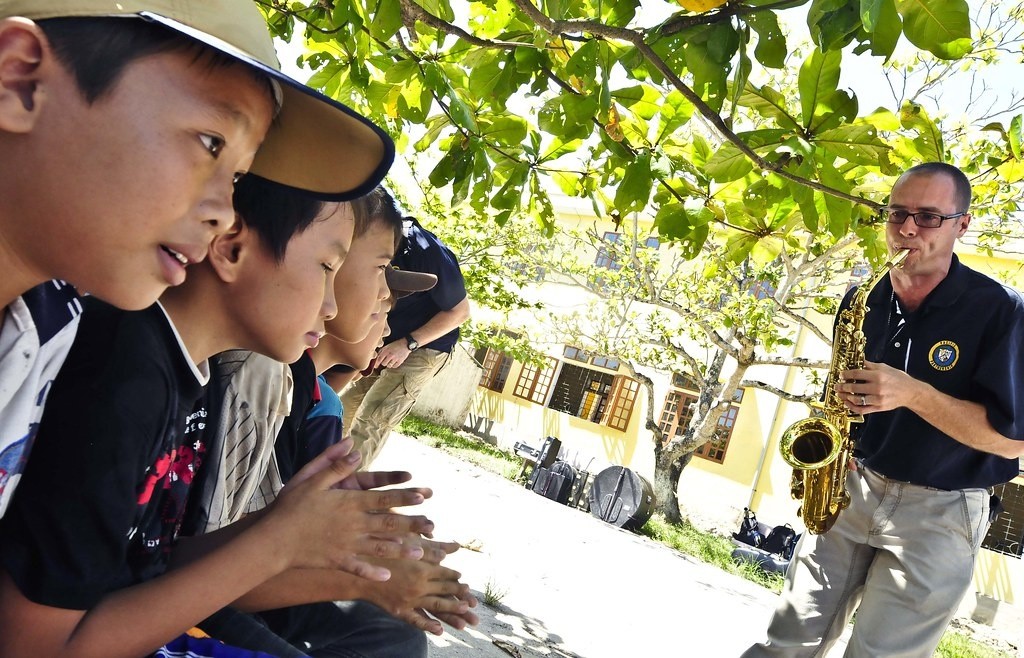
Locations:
731,509,802,561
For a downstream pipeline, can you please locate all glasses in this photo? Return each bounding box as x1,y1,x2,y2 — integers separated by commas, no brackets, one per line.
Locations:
879,206,965,229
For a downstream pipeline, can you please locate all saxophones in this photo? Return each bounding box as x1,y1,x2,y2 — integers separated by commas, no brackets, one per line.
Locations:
776,250,914,538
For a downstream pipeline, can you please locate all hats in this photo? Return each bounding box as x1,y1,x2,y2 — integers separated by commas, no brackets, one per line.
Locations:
385,261,440,301
1,0,396,203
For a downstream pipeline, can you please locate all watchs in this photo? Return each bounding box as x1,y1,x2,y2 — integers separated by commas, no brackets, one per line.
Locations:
405,333,418,352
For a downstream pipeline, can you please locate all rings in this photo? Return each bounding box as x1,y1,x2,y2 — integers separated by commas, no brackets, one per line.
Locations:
860,395,866,407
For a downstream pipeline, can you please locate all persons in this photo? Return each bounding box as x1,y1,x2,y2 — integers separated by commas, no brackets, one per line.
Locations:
741,162,1024,657
0,0,478,658
337,218,470,469
